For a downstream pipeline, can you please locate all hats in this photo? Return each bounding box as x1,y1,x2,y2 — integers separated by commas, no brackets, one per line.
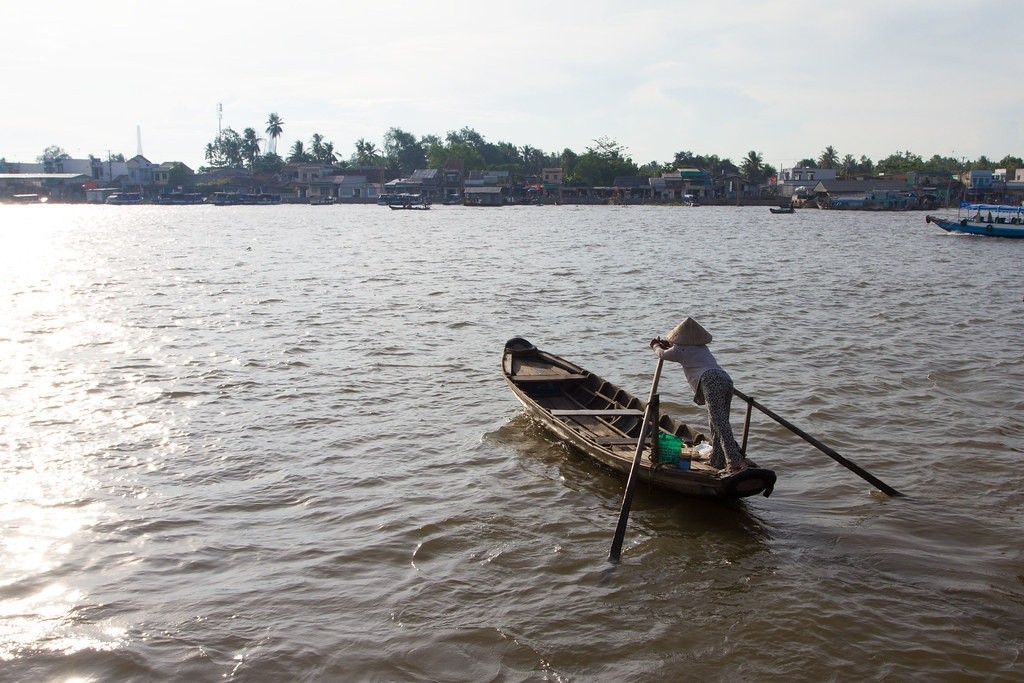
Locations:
666,317,712,346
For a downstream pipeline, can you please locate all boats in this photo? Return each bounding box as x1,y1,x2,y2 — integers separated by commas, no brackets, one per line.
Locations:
926,202,1024,238
158,194,203,204
769,207,796,214
309,194,334,205
389,203,429,210
377,193,423,205
502,335,776,499
106,191,142,204
213,193,281,205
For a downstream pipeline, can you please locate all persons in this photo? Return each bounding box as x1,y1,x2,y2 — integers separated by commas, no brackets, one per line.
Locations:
650,316,744,474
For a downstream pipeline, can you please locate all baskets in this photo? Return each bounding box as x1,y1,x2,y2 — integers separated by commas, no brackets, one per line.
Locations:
659,433,685,462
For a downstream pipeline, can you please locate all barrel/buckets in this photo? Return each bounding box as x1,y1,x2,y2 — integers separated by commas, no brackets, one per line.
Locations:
657,433,684,464
